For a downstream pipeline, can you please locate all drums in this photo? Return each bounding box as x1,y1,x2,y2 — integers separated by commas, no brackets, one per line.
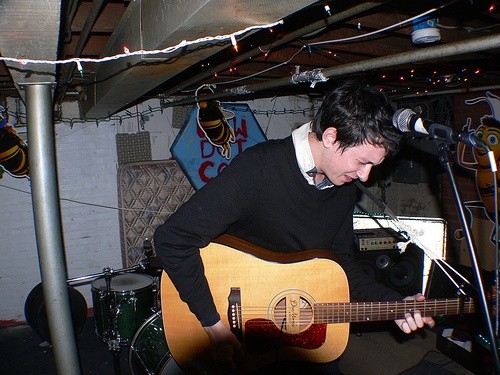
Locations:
128,307,183,375
90,270,158,353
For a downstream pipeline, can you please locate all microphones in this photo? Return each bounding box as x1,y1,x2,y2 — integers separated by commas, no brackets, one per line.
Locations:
392,108,489,152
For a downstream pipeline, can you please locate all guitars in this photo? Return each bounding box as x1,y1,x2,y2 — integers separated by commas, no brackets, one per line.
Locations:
159,232,481,375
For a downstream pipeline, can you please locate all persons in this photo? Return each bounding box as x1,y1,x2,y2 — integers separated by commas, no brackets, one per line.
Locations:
152,82,436,375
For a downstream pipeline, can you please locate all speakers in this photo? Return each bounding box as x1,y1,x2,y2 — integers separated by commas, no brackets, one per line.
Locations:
351,227,424,299
